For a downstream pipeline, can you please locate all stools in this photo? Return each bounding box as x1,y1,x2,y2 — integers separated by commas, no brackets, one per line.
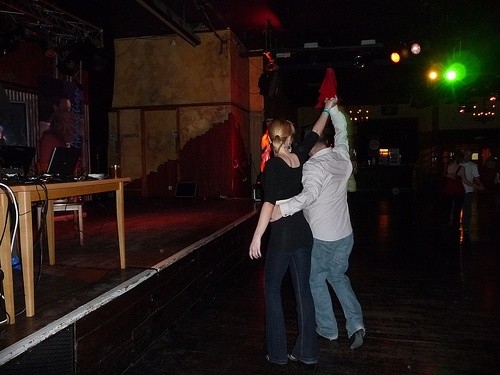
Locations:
35,202,83,248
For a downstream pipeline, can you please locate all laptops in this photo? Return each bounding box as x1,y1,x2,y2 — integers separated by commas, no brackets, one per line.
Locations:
0,144,37,178
43,147,82,177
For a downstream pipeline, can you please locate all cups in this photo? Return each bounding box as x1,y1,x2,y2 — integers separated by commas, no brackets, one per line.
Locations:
108,166,121,178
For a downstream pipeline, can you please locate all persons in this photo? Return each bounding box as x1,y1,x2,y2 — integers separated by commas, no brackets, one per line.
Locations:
249,98,335,364
269,95,366,349
444,151,480,231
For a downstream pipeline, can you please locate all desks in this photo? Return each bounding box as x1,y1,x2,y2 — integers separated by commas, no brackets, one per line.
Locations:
0,177,131,323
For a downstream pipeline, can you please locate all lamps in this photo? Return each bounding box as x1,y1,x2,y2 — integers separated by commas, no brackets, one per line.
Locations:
390,51,401,63
410,40,424,55
0,18,80,72
262,50,278,71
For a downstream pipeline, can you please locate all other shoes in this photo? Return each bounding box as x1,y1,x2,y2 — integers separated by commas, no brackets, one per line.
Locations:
315,333,337,344
464,227,468,233
287,353,318,364
12,254,22,271
349,329,364,349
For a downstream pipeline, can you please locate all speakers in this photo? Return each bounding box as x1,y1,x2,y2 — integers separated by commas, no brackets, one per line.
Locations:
259,71,279,97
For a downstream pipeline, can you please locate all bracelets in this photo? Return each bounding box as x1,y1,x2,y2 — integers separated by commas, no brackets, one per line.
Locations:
323,108,330,114
